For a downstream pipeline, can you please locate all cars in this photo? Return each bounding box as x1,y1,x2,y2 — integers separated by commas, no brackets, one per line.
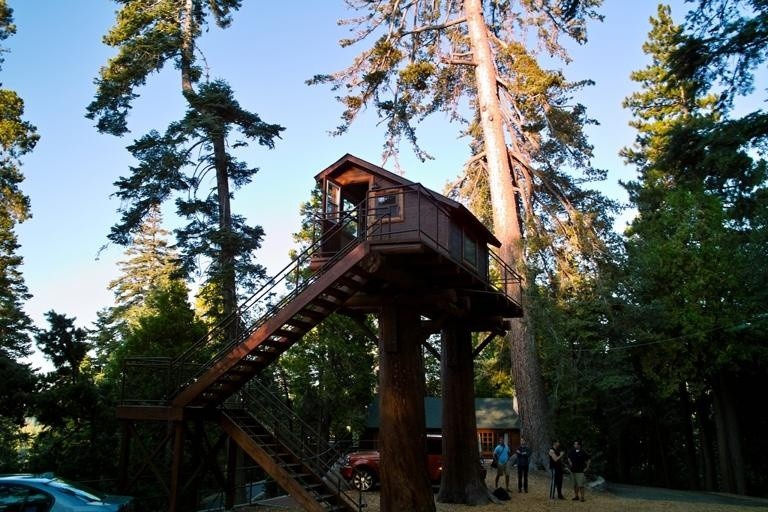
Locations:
0,473,135,512
340,434,487,491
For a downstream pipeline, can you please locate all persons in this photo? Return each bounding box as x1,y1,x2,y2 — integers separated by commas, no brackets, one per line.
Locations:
547,439,573,500
515,436,533,493
493,436,513,493
568,441,592,502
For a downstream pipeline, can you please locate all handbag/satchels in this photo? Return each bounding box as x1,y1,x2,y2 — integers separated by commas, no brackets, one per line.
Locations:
490,458,499,468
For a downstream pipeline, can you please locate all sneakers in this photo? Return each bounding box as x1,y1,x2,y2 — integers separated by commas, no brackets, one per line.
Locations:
573,496,584,502
550,494,563,499
507,487,528,493
